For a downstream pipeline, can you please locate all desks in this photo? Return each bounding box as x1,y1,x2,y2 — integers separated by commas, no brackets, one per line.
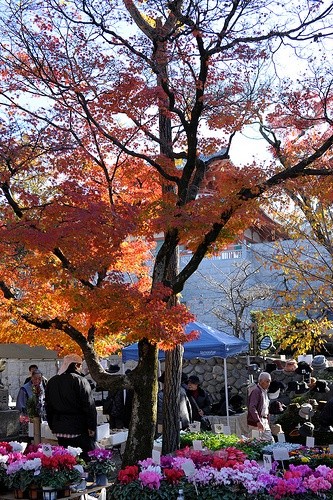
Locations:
209,410,250,436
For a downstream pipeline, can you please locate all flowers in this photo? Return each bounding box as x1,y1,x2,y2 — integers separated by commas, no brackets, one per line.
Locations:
0,415,333,500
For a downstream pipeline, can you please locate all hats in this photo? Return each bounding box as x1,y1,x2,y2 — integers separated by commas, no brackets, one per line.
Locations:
105,365,120,373
312,355,326,366
58,353,82,376
267,374,333,437
284,361,297,372
185,375,199,384
296,361,313,374
264,364,278,373
248,365,259,376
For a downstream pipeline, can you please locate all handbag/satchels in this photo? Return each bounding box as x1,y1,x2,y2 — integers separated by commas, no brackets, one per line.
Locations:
202,417,211,431
103,399,114,414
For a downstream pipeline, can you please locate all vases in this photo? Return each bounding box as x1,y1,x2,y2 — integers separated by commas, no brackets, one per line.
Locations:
96,473,108,486
61,486,72,496
28,483,38,500
13,487,24,499
42,485,57,500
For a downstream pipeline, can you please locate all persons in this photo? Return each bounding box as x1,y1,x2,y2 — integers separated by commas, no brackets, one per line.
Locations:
16,362,245,441
46,353,97,459
247,372,275,442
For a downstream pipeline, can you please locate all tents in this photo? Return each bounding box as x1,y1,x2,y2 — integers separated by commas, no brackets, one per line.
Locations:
120,319,250,426
